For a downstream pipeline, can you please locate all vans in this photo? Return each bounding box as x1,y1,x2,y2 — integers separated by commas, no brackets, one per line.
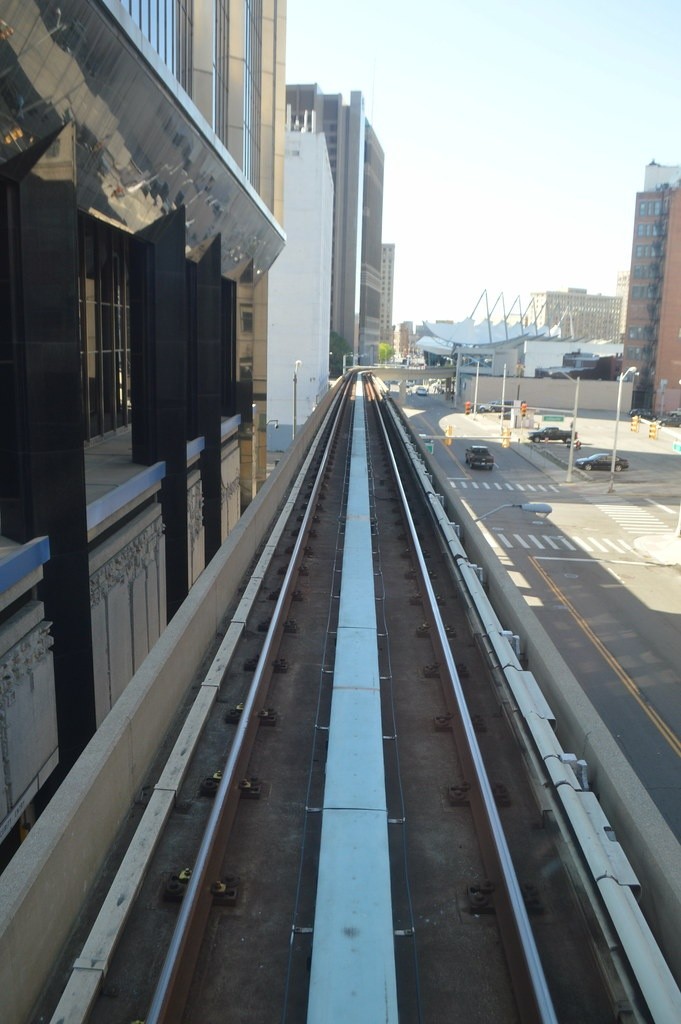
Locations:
488,400,525,413
659,417,681,427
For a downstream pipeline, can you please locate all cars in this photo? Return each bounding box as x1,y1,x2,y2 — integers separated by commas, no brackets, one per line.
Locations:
575,453,630,471
628,407,681,421
417,387,427,396
478,401,500,413
398,379,425,393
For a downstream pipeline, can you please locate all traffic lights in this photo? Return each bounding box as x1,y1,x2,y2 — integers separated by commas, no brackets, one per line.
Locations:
630,415,640,433
648,423,660,441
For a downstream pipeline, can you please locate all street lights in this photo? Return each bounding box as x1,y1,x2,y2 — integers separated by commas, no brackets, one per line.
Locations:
473,503,553,523
549,369,580,484
460,354,480,422
267,419,280,429
484,358,507,435
292,360,301,438
608,366,638,493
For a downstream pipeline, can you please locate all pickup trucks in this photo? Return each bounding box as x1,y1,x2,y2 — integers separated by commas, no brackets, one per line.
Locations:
528,426,578,443
465,445,495,471
